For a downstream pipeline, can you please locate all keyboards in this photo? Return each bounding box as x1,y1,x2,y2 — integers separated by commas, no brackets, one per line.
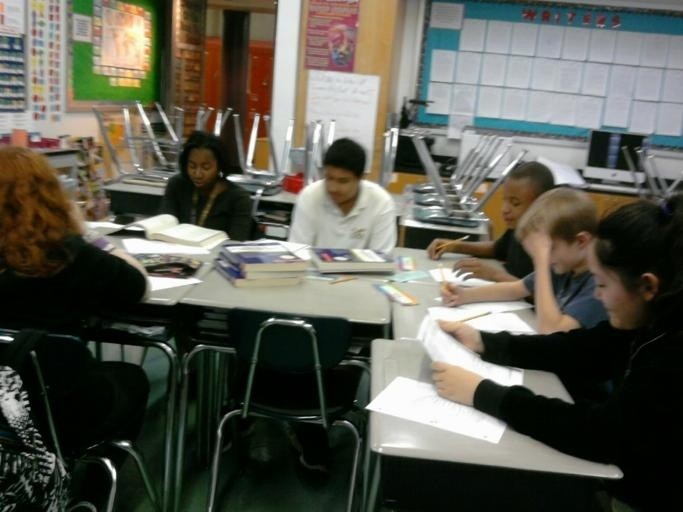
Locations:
591,184,639,195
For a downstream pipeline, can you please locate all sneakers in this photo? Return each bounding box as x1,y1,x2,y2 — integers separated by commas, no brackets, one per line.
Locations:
298,429,326,472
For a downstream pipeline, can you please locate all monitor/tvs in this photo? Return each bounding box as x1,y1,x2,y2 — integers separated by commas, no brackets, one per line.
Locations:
582,128,648,184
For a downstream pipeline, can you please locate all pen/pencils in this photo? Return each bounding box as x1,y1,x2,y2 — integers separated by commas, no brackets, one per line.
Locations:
458,312,491,321
436,235,470,250
439,266,448,290
329,278,359,284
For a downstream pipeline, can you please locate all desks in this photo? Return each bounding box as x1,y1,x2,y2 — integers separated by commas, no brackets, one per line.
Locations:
392,243,512,282
389,277,553,349
64,216,232,444
369,331,627,497
182,227,389,478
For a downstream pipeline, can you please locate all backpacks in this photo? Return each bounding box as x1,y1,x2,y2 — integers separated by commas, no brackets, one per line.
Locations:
0,331,77,512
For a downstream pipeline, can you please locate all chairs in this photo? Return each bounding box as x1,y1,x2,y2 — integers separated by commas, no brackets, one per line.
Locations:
204,307,371,510
84,100,677,246
1,326,161,509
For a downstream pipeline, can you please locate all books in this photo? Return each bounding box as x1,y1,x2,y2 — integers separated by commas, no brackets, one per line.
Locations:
310,246,396,274
134,253,204,279
108,213,232,250
212,239,307,289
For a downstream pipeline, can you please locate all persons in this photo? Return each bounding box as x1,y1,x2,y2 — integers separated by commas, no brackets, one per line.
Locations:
443,187,608,334
428,193,683,511
1,146,152,512
427,162,553,310
159,129,250,400
287,137,400,254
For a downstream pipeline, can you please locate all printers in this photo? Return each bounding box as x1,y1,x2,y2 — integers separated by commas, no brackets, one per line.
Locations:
458,128,516,179
